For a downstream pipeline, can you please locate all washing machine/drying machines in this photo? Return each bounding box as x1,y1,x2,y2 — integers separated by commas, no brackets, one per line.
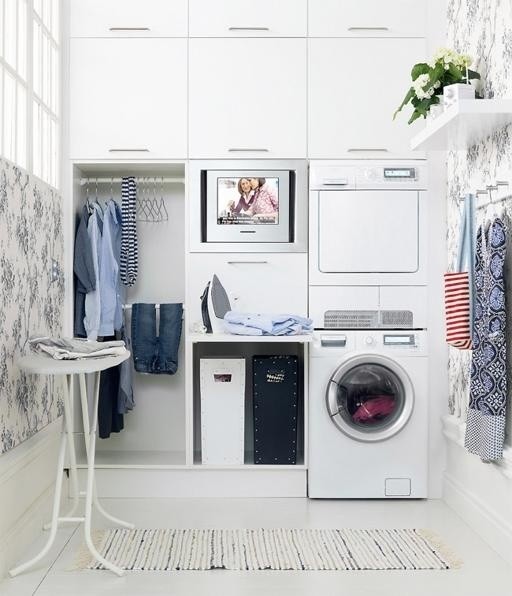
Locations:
306,330,428,501
307,160,429,329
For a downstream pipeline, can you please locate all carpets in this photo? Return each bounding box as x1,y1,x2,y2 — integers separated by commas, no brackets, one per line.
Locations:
74,526,462,572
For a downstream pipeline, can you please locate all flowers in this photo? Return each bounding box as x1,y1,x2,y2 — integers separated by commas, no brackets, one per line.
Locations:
392,49,485,124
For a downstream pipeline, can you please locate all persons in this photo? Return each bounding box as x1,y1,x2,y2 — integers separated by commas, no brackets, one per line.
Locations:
243,177,279,216
226,177,256,215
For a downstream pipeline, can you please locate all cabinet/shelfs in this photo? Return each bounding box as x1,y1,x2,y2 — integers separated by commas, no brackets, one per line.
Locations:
187,1,307,160
71,2,189,160
308,3,427,159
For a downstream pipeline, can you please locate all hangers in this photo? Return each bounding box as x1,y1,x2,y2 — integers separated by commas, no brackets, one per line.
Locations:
83,177,169,222
480,184,504,225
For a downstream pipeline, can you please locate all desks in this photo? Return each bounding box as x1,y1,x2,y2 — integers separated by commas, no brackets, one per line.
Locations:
8,354,134,578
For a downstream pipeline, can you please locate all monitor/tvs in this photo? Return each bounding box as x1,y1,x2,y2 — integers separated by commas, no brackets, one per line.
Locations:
206,171,290,243
